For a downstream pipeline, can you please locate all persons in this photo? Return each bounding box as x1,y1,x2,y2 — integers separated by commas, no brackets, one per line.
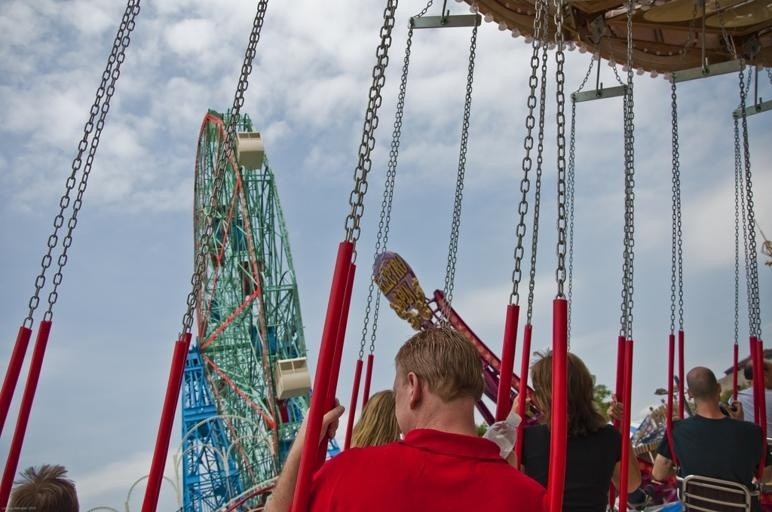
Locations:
260,327,549,512
481,350,644,512
727,358,772,445
351,389,404,448
5,462,82,512
649,363,767,512
